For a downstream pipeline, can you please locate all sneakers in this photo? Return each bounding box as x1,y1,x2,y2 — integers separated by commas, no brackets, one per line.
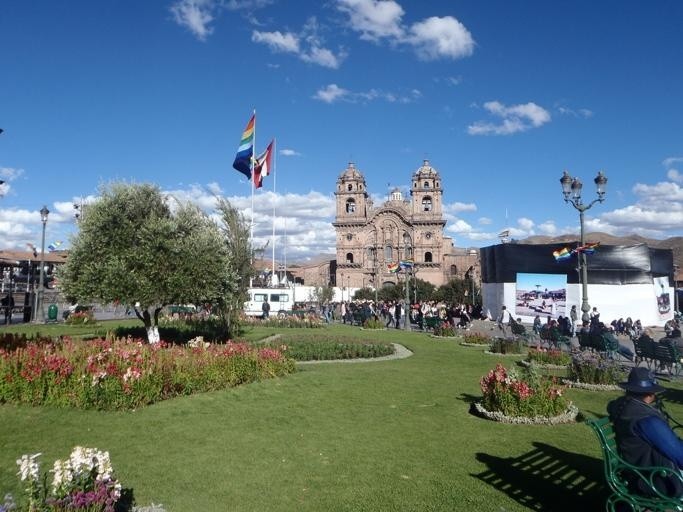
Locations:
458,323,473,332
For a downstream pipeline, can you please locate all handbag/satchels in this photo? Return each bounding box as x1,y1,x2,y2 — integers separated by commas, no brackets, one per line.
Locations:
508,313,513,326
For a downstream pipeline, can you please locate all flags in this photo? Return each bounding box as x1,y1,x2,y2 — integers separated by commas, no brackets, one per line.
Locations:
551,247,573,264
387,263,402,273
399,257,415,269
232,115,255,181
571,242,600,257
253,142,273,189
47,239,64,251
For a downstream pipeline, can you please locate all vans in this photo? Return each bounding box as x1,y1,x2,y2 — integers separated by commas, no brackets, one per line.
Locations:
237,286,295,320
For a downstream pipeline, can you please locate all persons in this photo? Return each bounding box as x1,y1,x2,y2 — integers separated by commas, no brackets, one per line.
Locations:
638,319,682,360
2,293,15,324
262,300,270,320
293,298,492,330
610,366,683,498
498,305,642,355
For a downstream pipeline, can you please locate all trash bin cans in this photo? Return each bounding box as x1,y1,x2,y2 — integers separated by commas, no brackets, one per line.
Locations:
48,304,58,321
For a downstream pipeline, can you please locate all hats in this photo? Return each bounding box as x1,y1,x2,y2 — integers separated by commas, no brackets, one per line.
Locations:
617,366,667,395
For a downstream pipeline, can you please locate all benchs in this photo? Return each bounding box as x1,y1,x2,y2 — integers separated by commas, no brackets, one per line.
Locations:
284,310,445,333
585,399,683,512
169,307,194,318
511,324,682,378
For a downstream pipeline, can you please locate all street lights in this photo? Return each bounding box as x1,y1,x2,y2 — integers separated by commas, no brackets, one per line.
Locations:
30,204,48,324
400,232,413,330
558,168,607,333
